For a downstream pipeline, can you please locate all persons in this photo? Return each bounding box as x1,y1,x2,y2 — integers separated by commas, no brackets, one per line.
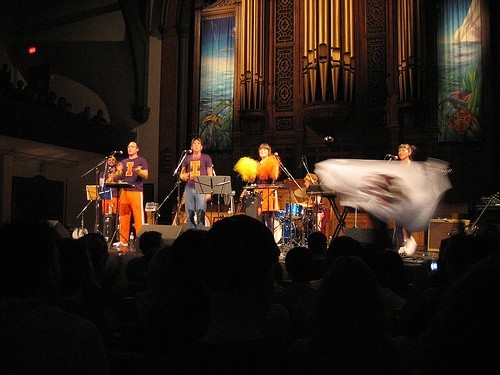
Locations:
257,144,278,233
303,173,324,233
395,144,417,256
110,142,148,246
1,214,500,375
179,137,214,230
0,68,107,127
99,156,118,241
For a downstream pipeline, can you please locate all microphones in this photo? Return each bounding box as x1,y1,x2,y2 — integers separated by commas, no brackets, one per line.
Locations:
299,157,304,168
184,150,192,153
113,150,123,154
387,154,398,160
273,153,278,158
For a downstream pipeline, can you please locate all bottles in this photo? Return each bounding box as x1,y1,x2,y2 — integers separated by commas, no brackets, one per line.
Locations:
130,232,134,244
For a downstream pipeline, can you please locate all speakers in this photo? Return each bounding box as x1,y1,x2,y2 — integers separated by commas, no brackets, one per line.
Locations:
136,225,183,254
345,227,403,249
427,219,469,252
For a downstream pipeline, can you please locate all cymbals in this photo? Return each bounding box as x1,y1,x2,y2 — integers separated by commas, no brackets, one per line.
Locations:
243,184,290,189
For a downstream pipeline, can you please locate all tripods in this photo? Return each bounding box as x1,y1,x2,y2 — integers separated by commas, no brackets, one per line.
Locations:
106,155,135,252
271,156,329,259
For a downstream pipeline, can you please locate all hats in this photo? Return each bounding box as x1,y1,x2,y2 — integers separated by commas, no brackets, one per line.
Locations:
108,155,116,161
191,137,201,145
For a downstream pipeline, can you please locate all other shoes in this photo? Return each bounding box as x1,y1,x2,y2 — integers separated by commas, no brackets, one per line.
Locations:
112,241,128,247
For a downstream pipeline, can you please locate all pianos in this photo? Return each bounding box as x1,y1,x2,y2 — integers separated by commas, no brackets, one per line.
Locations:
305,183,351,249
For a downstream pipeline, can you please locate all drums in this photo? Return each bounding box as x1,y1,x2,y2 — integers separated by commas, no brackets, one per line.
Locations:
261,209,287,221
301,202,320,240
285,202,303,221
268,215,302,246
294,187,309,199
